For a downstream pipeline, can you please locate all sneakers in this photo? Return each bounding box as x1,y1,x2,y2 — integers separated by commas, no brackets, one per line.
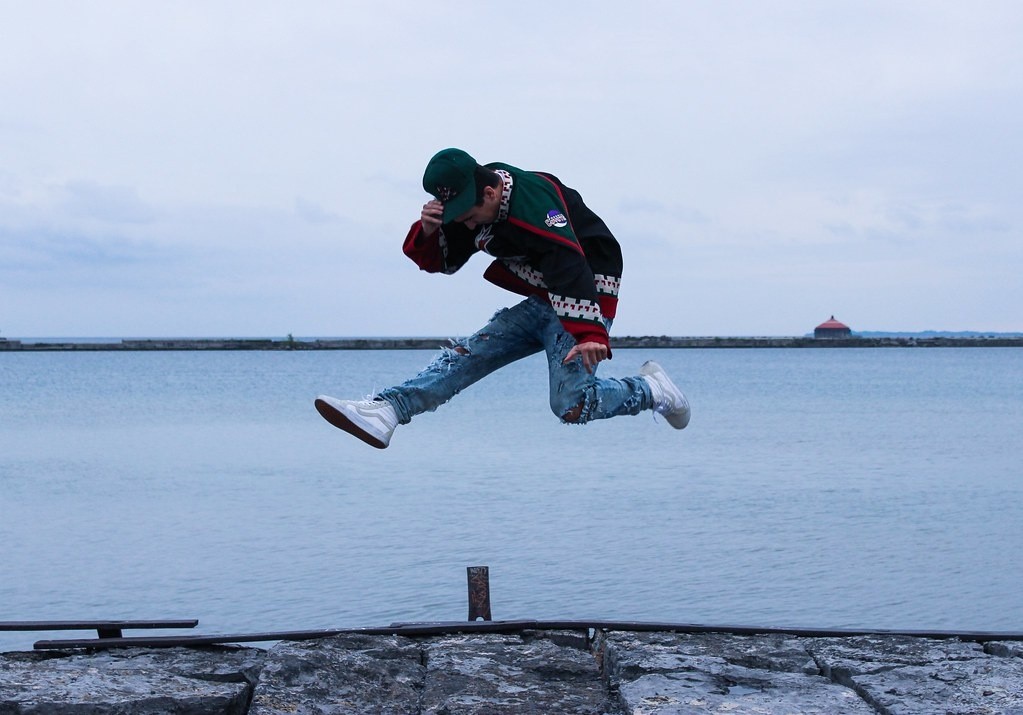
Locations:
314,382,399,449
639,360,691,430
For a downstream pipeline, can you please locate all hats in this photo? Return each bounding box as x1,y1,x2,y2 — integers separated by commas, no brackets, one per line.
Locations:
423,148,479,224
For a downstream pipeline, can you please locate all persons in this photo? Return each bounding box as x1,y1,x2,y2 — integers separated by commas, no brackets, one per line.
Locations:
314,148,692,450
